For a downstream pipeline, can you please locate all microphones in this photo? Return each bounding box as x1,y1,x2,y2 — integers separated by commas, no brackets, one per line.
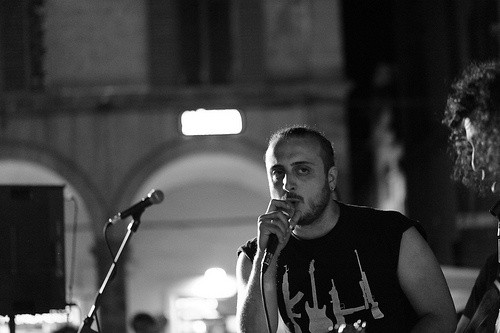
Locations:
260,207,295,272
109,189,164,225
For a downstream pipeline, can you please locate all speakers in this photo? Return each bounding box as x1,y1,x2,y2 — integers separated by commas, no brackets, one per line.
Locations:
0,185,65,316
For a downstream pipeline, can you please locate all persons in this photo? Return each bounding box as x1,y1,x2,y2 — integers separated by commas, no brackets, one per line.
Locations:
443,62,500,333
236,126,458,333
